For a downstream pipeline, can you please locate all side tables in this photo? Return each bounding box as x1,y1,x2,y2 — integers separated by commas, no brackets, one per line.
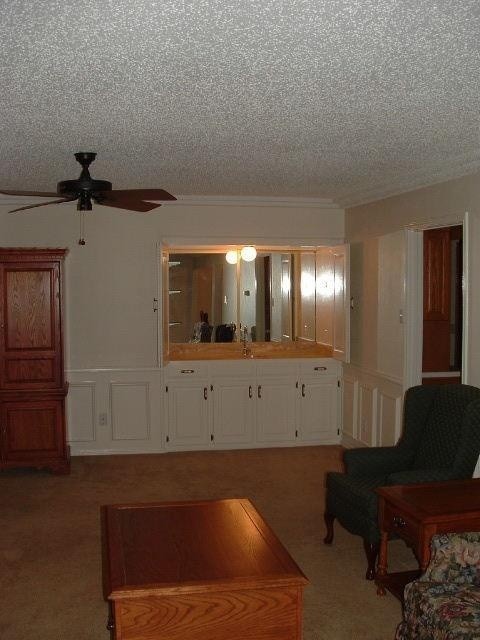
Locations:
371,477,479,603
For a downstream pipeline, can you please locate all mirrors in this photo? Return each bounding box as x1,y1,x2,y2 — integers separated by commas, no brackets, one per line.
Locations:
163,244,334,361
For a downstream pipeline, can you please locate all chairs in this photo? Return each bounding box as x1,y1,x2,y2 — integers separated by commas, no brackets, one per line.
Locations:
323,382,479,581
398,532,478,636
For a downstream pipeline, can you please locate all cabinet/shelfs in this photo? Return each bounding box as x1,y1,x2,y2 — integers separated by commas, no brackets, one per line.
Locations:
163,362,210,451
298,362,342,447
0,248,72,475
211,362,298,451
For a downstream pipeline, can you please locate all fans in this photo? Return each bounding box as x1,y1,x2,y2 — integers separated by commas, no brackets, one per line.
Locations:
1,150,179,217
